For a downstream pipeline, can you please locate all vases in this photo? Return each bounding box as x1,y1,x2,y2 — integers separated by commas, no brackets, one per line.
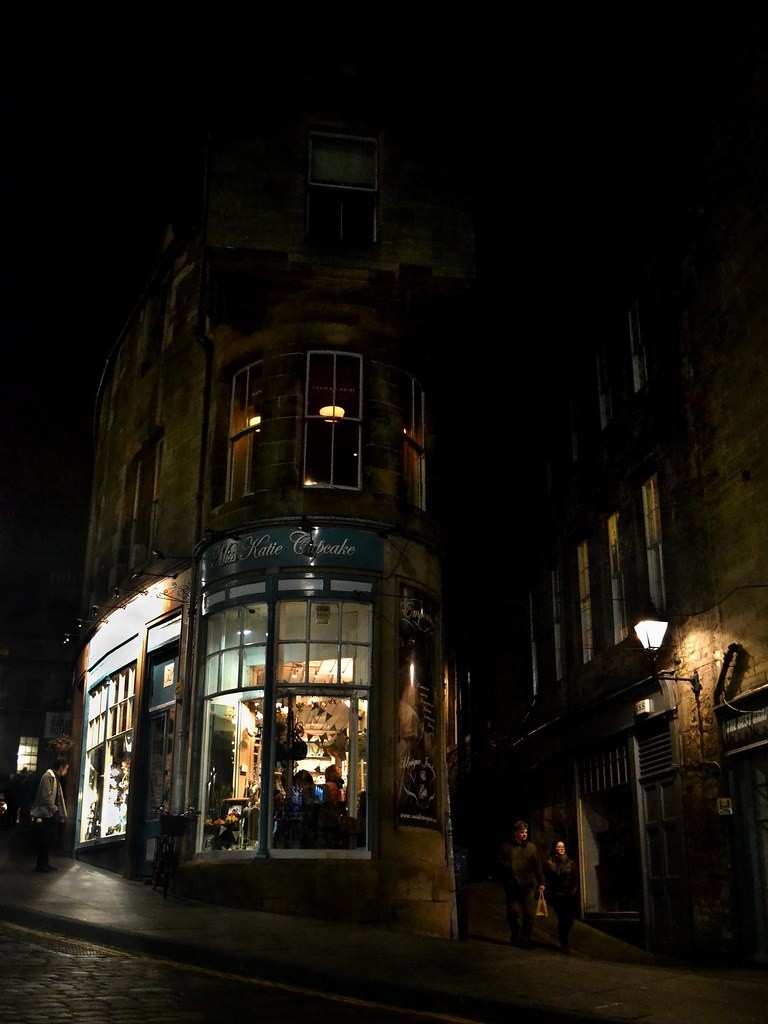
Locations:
51,742,69,752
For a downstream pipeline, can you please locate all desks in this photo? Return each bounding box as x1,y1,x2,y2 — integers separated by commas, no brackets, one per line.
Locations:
219,797,250,849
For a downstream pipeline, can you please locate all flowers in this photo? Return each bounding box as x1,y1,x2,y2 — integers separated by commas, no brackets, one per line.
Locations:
45,732,74,746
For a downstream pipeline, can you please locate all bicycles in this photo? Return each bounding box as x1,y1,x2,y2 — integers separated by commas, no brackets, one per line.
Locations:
144,805,202,898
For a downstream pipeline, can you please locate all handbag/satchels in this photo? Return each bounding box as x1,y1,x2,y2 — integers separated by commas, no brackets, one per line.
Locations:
535,890,548,917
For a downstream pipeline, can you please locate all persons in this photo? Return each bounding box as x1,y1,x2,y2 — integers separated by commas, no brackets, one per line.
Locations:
30,757,70,874
541,840,580,956
275,765,350,852
496,820,548,949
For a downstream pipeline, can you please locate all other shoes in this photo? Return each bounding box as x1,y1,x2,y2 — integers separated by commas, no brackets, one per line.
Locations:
510,929,520,943
46,863,58,870
559,936,568,947
35,865,51,873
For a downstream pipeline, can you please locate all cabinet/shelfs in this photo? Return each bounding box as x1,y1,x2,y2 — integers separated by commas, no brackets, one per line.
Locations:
258,729,343,795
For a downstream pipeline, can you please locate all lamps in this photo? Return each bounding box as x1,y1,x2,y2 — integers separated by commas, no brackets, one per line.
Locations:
297,519,315,544
377,524,416,541
203,526,241,545
60,548,203,647
633,595,703,696
318,404,346,423
